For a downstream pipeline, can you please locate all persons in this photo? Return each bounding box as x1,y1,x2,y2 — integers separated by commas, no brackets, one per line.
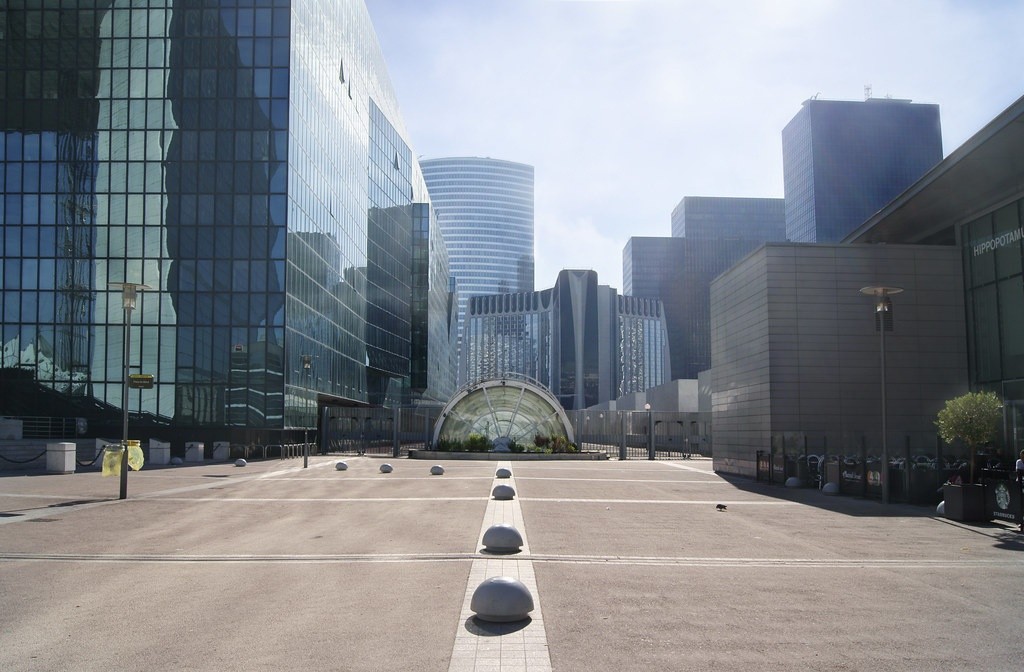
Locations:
1015,449,1024,505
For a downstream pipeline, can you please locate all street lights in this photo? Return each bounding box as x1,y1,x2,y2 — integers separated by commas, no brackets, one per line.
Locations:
107,281,153,498
859,284,905,505
299,354,319,468
